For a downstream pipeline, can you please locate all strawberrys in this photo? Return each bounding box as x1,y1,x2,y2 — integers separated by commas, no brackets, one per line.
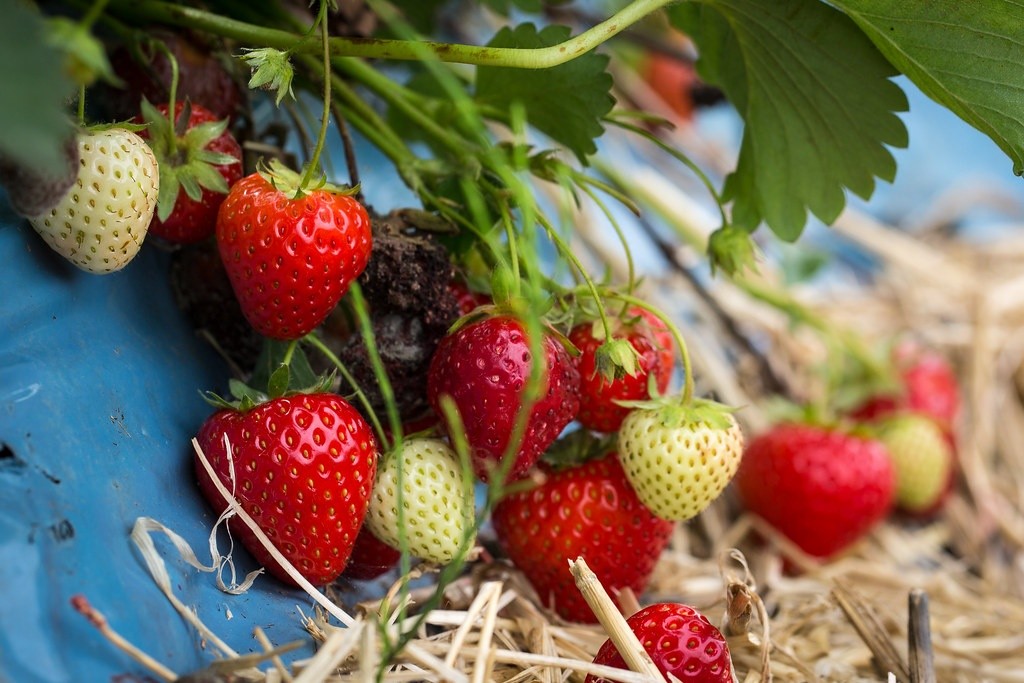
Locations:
22,57,957,683
